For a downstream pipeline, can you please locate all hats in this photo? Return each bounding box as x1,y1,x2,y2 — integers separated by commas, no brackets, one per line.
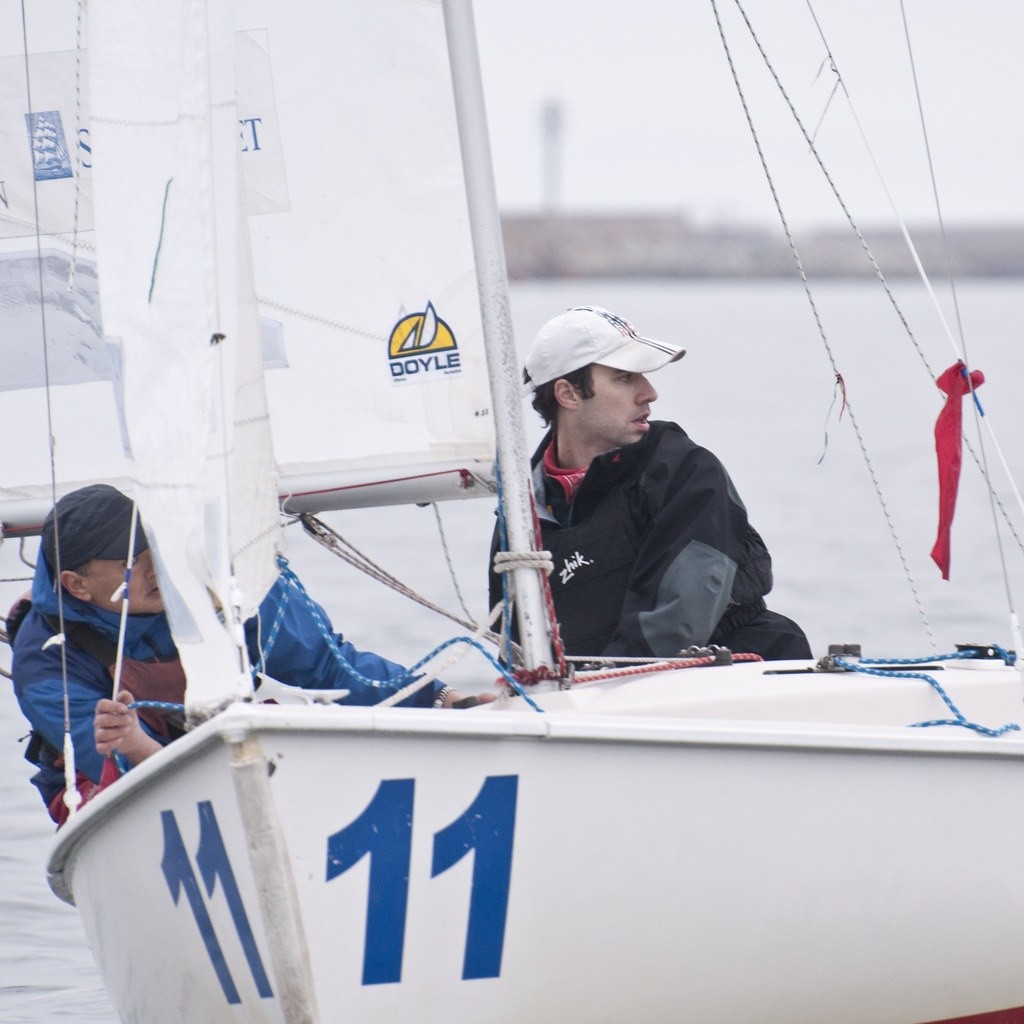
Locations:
521,306,686,399
42,484,149,578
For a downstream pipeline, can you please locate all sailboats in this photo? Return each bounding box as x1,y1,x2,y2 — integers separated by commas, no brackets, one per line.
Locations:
0,0,1022,1024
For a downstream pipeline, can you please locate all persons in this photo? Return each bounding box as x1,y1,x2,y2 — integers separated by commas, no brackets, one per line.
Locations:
11,483,499,832
487,307,813,660
